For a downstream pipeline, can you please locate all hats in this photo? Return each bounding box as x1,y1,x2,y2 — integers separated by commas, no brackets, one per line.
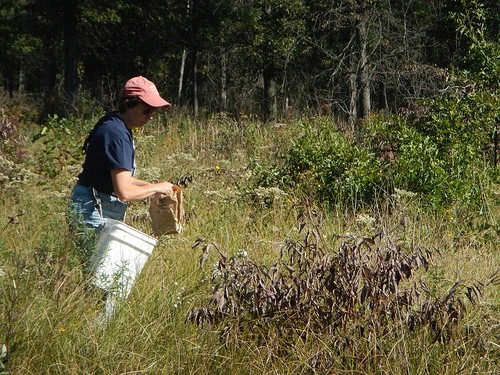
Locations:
121,74,171,110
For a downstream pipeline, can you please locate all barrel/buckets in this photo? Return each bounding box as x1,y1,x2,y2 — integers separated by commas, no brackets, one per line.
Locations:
84,218,158,302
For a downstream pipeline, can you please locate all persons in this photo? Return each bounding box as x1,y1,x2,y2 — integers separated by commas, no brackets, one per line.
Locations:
65,74,173,267
